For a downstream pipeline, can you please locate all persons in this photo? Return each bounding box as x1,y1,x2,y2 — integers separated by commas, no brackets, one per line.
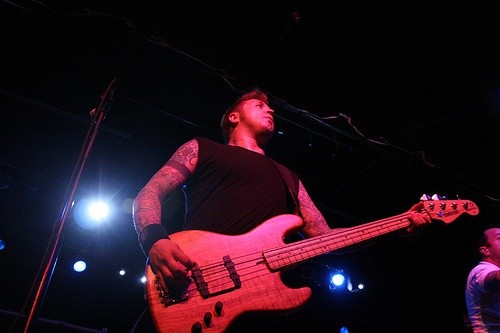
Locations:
132,85,433,331
466,222,500,333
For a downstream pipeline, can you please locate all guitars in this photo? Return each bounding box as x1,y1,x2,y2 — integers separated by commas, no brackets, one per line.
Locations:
144,193,479,332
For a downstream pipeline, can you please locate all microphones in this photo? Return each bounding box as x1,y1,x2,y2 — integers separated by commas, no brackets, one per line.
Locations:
89,82,118,118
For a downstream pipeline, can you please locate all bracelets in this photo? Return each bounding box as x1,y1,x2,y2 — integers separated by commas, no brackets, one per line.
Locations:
138,222,171,258
